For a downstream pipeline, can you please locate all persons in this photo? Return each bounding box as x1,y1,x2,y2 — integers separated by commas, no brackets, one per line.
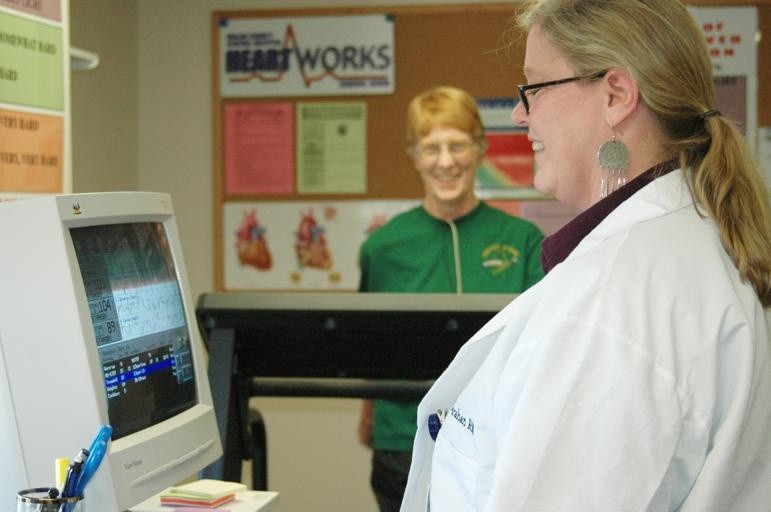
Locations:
360,83,547,512
396,0,771,512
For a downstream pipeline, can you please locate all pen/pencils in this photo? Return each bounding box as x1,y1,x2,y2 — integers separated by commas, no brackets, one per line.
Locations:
37,424,113,512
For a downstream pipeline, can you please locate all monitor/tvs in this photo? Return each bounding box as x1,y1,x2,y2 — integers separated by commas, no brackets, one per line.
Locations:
0,191,223,512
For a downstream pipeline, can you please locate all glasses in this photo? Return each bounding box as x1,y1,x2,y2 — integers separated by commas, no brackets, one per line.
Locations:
515,72,604,113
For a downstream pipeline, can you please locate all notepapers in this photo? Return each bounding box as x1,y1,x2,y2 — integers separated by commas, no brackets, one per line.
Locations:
159,487,235,512
